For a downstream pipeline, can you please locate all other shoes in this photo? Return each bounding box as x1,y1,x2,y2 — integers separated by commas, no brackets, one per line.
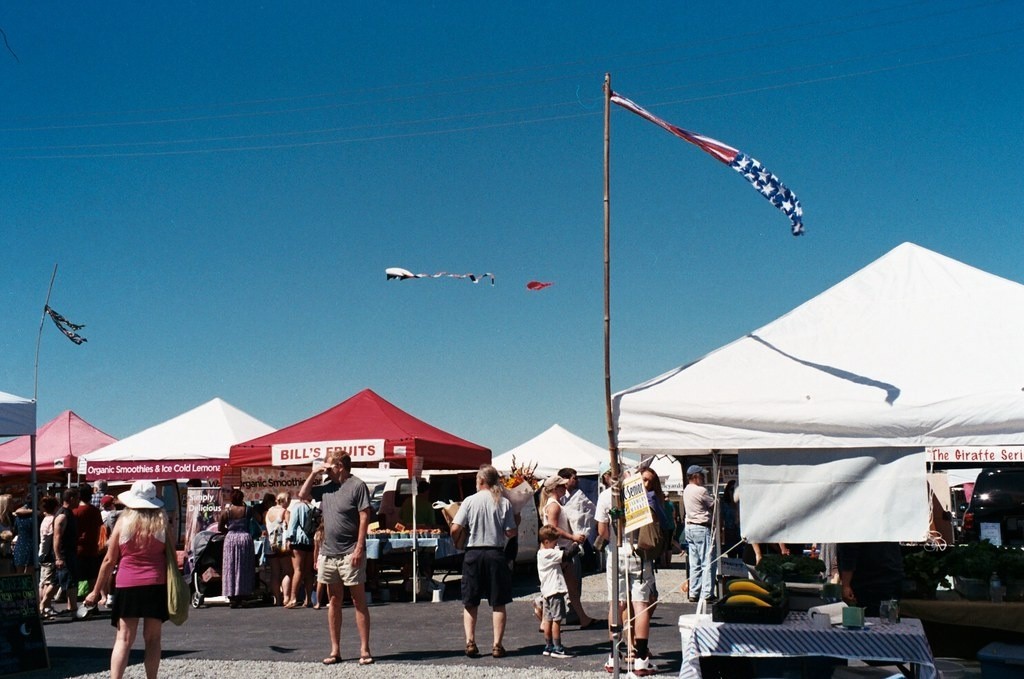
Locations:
57,608,78,617
105,595,114,607
283,601,299,609
44,606,58,616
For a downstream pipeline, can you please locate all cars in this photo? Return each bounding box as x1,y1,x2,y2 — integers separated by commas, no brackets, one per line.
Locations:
965,466,1024,548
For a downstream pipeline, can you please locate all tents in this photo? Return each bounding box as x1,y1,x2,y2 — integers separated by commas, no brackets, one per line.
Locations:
0,389,42,581
0,410,120,489
606,236,1024,679
487,419,637,480
207,387,494,609
72,397,280,482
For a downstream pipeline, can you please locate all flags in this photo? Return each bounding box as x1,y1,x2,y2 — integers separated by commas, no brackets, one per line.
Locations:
609,90,808,236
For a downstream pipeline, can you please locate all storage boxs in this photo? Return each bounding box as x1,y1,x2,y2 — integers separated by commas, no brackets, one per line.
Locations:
976,636,1024,679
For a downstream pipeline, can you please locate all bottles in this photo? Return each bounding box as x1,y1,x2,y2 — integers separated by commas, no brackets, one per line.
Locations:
891,599,900,624
990,571,1002,602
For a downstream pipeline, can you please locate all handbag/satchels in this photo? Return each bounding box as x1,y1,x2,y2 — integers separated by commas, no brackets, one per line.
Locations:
97,523,109,555
166,528,190,627
39,533,55,566
431,498,468,550
270,507,287,555
637,519,663,560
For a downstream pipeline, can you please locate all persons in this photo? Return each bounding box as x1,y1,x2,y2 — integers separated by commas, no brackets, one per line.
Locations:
682,465,719,604
725,465,948,618
537,475,602,634
84,483,183,678
296,449,376,666
589,462,681,674
1,477,379,619
439,463,523,661
529,524,587,661
531,467,580,624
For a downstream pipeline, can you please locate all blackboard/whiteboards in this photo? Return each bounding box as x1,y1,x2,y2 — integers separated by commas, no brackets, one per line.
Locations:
0,572,52,678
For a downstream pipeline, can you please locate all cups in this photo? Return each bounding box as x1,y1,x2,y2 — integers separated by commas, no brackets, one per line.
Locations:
880,600,896,625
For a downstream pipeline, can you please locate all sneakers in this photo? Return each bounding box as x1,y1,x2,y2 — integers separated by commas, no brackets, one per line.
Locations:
604,653,621,673
492,646,507,658
465,641,479,654
543,646,577,658
633,657,659,676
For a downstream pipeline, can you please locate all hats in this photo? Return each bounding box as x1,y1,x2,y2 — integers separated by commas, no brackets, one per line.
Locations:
686,465,703,474
544,475,569,491
101,493,114,508
117,481,165,509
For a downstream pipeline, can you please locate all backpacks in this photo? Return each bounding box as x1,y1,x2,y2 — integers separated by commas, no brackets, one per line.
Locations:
299,502,322,544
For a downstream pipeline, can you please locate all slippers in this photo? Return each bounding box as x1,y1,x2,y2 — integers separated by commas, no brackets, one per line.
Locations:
324,655,342,664
359,656,374,665
580,618,608,630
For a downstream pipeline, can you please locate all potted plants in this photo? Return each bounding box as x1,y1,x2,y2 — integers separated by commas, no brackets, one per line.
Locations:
901,537,1024,603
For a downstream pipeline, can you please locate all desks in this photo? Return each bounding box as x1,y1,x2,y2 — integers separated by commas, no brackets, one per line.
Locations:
678,613,937,679
898,598,1024,633
362,538,466,598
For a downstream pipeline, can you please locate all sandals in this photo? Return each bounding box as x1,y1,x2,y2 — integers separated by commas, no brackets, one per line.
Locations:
40,611,55,620
